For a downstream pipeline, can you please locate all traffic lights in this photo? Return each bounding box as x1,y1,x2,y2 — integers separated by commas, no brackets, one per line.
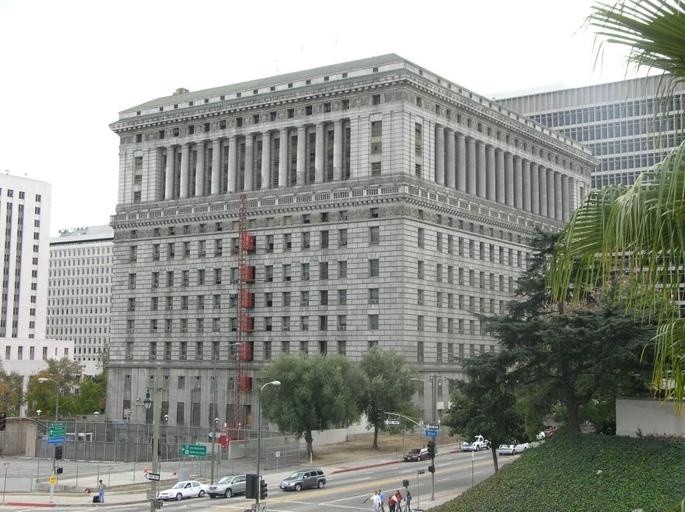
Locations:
0,412,7,431
427,441,435,459
260,479,268,501
55,446,63,460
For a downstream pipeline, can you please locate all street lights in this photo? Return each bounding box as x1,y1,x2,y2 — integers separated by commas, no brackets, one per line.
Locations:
143,366,169,512
37,376,59,504
256,380,282,505
429,373,443,421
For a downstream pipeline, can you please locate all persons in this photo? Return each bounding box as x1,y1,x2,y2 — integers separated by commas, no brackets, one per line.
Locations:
97,479,106,502
370,488,413,512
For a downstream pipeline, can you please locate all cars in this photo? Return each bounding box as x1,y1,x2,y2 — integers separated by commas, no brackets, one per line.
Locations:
279,468,327,492
498,440,529,456
403,447,432,462
158,479,209,502
462,434,492,452
206,474,246,499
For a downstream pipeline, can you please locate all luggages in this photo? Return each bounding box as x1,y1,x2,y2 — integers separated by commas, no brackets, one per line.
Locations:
93,495,99,503
